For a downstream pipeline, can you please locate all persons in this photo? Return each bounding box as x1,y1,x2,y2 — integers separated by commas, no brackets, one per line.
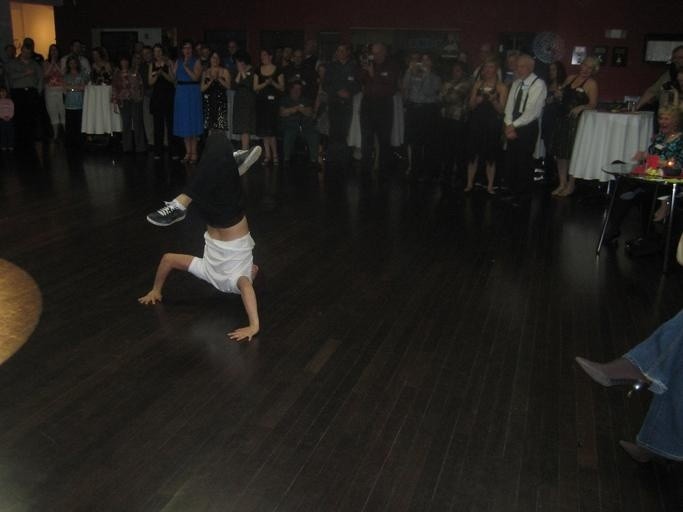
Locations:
659,61,682,108
594,103,683,252
572,305,682,463
634,45,683,112
136,131,263,341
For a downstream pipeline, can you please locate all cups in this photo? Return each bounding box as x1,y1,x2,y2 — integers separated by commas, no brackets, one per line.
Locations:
623,95,640,115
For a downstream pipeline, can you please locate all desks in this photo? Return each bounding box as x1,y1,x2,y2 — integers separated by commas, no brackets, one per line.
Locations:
568,109,654,195
595,163,683,272
80,85,123,137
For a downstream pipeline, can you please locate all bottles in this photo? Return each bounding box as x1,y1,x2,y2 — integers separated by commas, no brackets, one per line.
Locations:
636,144,666,178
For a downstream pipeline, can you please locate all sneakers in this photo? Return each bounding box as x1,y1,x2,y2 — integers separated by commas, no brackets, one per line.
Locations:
146,199,187,227
232,145,263,177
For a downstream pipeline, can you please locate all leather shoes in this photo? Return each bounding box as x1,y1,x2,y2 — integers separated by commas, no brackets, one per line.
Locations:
189,153,199,164
180,153,191,164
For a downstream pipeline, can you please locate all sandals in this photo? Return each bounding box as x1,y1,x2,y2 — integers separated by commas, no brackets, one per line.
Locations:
261,157,271,166
272,156,280,166
